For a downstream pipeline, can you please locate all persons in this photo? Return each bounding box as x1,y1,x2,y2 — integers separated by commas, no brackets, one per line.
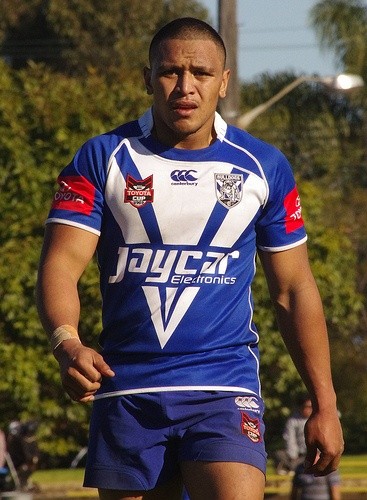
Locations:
0,425,9,474
3,419,39,493
281,392,313,471
290,445,343,500
34,18,347,500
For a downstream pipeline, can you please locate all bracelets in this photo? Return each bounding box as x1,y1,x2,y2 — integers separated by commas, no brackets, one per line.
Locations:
49,324,82,358
